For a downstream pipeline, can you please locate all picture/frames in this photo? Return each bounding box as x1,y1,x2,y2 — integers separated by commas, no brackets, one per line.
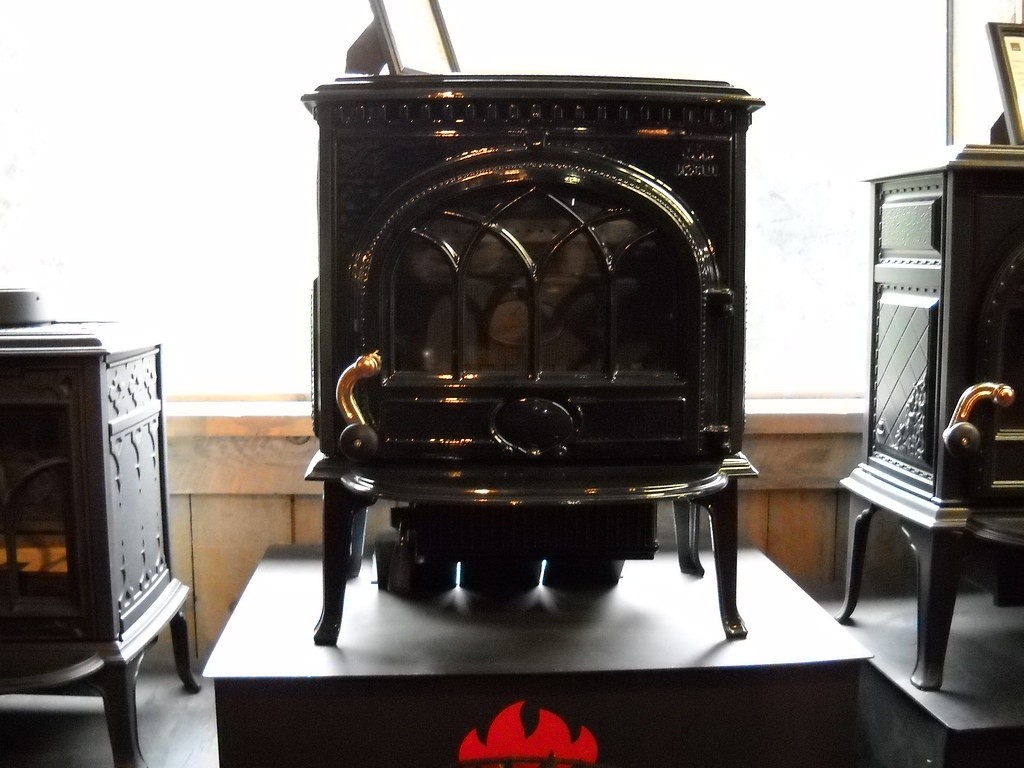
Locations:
986,21,1024,147
345,0,461,75
